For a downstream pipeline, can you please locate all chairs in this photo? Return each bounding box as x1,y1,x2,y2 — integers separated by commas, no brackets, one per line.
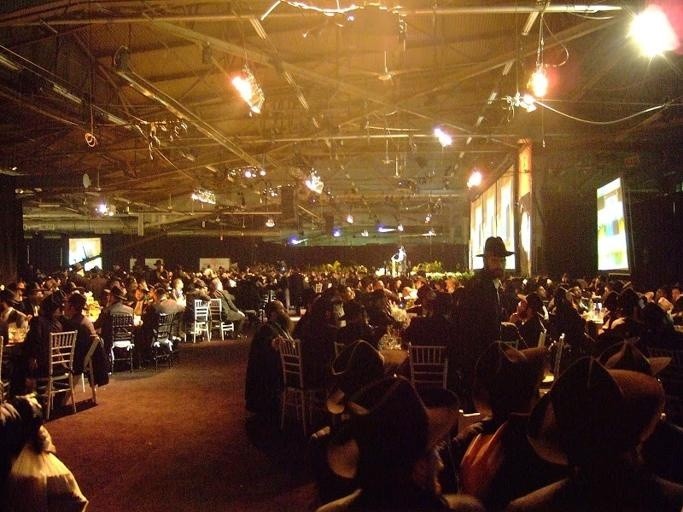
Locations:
542,334,565,385
169,311,182,348
153,314,176,370
184,300,208,345
0,336,10,401
35,330,78,418
208,299,234,340
111,314,133,373
60,337,101,406
408,343,450,390
277,337,331,436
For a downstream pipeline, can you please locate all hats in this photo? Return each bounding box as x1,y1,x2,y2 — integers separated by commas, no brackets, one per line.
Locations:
524,355,617,472
630,303,666,335
306,373,461,493
337,298,371,323
325,338,409,416
516,290,547,316
42,288,67,316
66,293,90,312
8,281,27,291
153,258,166,268
414,284,441,306
103,285,129,301
0,286,21,306
295,291,323,309
475,236,516,258
595,340,673,378
70,262,85,274
462,339,549,406
192,278,207,290
667,281,683,289
27,281,47,292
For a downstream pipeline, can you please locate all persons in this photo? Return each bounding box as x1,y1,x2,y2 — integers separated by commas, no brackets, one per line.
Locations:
460,236,517,414
0,403,52,454
315,374,485,512
451,339,565,512
596,344,683,485
502,273,682,361
309,339,460,512
246,301,296,431
297,262,471,383
388,239,410,270
0,260,243,410
507,358,683,512
243,259,297,328
